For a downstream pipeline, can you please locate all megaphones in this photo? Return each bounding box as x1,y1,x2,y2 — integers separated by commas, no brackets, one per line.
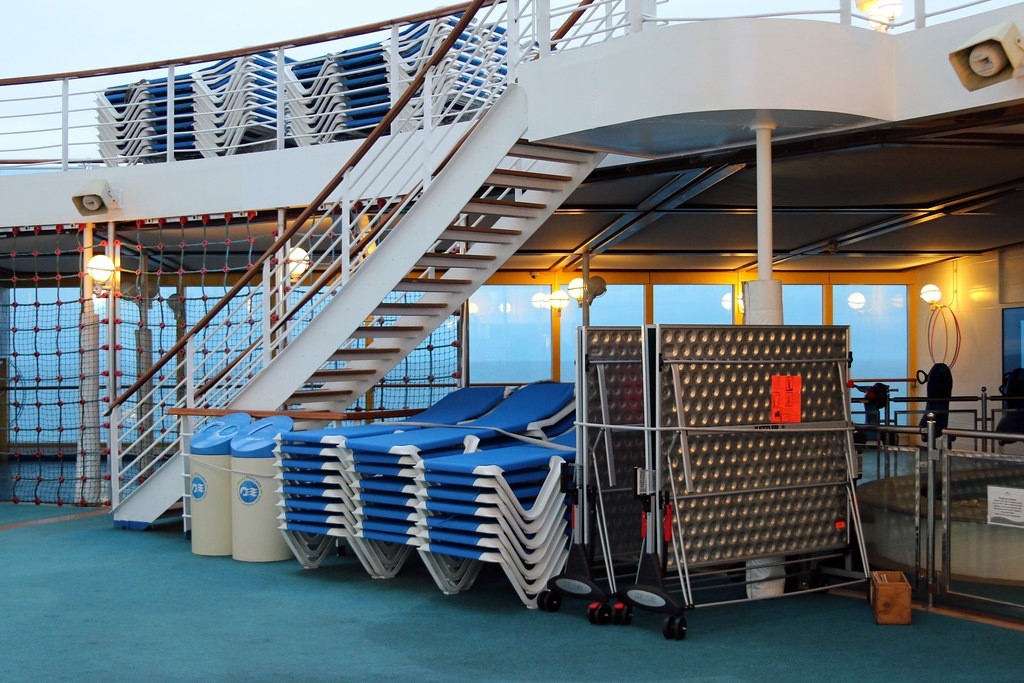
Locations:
949,21,1024,92
73,179,113,217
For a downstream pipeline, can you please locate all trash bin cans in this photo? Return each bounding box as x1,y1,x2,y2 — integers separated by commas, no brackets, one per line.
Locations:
189,412,252,557
230,414,296,562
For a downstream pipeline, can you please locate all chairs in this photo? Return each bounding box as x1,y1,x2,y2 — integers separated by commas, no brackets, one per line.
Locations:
272,381,579,618
96,11,558,168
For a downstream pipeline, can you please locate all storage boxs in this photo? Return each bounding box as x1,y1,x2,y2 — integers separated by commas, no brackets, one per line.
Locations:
870,571,912,625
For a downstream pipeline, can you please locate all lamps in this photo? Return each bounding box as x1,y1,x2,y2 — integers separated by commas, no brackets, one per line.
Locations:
289,248,309,287
550,291,569,317
856,0,903,34
568,278,586,307
168,294,182,317
121,281,141,308
584,276,607,306
142,279,160,306
920,261,955,312
847,285,878,314
87,255,114,295
